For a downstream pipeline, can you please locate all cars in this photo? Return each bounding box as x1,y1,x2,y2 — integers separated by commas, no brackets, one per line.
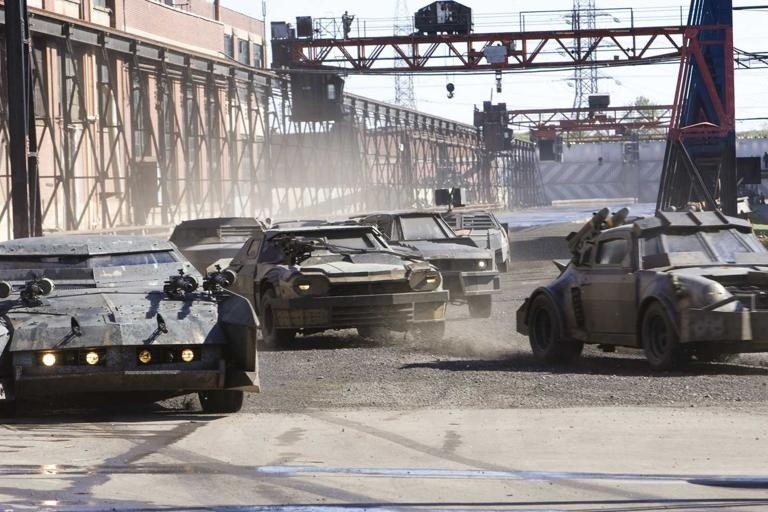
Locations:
516,208,768,371
0,237,261,420
